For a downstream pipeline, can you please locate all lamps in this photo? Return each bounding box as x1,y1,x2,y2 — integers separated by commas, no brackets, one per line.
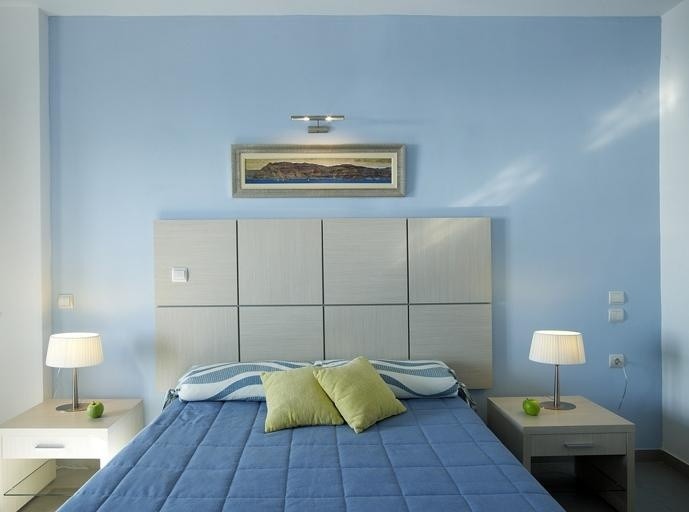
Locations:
290,114,345,135
527,330,587,412
45,332,105,414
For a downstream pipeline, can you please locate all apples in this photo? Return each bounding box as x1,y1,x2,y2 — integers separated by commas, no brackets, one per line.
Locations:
523,397,541,416
87,400,104,418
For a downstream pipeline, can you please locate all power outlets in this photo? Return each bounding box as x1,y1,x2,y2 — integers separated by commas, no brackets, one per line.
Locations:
607,353,626,369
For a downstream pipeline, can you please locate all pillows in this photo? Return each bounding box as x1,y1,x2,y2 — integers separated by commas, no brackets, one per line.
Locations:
314,359,478,407
162,358,309,411
257,364,343,435
311,355,408,435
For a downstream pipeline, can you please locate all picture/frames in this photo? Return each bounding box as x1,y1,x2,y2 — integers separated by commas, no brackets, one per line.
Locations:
229,143,407,198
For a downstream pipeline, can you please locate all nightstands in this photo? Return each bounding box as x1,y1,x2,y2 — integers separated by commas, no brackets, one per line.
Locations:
486,393,638,511
1,396,144,499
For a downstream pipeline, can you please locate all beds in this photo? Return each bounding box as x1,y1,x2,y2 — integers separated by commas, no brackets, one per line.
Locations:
54,218,566,512
